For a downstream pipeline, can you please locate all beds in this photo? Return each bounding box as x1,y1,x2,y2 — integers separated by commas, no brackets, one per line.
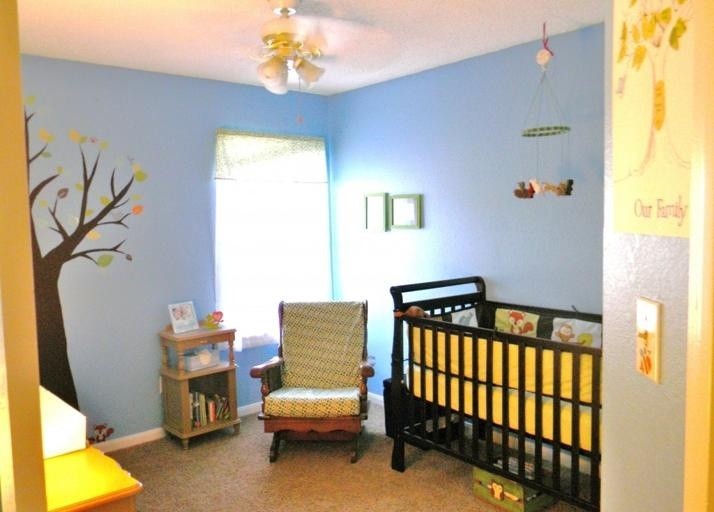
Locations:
389,276,603,512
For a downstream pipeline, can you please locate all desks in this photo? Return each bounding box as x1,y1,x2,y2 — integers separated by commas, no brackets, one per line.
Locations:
40,441,144,512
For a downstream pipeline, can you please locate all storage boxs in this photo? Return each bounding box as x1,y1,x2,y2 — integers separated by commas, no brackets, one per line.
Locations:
471,456,556,512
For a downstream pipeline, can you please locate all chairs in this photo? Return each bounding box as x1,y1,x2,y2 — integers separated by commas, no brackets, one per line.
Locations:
249,298,376,464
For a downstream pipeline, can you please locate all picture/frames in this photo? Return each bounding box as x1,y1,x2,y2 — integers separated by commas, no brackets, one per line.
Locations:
168,300,200,335
365,192,422,233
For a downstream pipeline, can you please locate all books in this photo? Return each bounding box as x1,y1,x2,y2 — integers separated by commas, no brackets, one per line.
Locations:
189,391,216,429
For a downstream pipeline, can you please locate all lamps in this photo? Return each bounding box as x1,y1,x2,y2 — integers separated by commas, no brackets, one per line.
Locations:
254,7,326,95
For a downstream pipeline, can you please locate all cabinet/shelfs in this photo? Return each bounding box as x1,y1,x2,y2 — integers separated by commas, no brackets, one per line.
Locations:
159,322,243,450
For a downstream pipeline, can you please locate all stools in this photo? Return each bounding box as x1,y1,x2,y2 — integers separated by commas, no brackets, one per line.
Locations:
383,374,464,451
